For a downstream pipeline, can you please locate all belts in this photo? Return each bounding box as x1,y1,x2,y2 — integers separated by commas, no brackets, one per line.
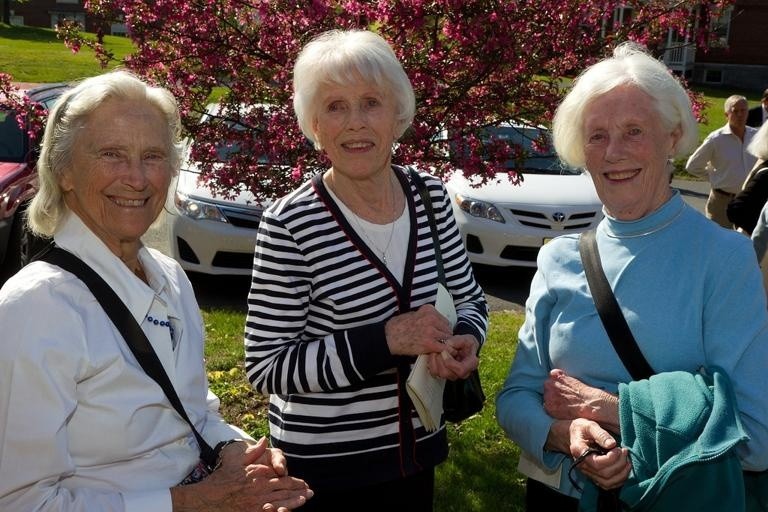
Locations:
714,188,735,198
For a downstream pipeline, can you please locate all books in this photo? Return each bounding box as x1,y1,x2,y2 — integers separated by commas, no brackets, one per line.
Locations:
405,283,455,429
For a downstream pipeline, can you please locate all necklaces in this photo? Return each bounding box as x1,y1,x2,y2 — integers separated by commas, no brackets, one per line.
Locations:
337,179,398,266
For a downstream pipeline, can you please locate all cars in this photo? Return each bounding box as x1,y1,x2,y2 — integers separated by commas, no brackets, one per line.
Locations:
0,82,75,274
413,113,605,269
165,101,328,277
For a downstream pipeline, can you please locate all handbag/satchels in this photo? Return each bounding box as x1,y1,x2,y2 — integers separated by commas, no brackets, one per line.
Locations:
442,370,485,425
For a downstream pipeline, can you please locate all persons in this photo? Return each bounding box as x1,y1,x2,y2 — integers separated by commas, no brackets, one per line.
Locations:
495,41,768,509
4,69,323,512
727,121,768,231
687,95,759,229
235,30,489,510
